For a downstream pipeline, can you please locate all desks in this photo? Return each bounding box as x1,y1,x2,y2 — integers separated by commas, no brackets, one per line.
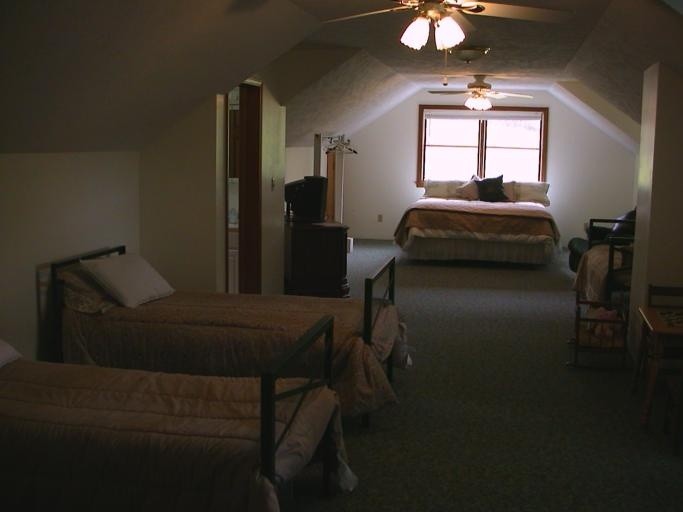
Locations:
631,305,682,427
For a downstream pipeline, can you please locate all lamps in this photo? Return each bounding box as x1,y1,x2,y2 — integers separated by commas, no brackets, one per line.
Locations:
398,16,466,52
464,96,492,111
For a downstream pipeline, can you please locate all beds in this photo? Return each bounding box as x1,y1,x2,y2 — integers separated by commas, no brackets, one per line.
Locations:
2,314,342,511
50,246,395,434
407,179,555,270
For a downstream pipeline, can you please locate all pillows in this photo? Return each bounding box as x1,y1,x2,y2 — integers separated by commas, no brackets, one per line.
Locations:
3,340,24,367
422,174,550,207
79,252,178,309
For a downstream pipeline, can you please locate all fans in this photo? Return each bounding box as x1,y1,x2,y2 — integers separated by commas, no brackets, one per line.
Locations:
322,1,571,87
427,75,534,111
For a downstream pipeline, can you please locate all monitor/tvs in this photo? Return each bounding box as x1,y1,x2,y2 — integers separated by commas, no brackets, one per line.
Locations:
285,174,329,224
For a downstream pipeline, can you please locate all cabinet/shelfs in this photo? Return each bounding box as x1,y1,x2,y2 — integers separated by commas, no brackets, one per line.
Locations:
284,219,350,298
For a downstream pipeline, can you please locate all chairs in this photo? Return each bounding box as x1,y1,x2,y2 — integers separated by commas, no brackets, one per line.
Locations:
632,284,683,393
575,218,635,301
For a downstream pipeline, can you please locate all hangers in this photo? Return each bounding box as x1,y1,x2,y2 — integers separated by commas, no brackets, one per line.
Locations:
323,136,358,156
